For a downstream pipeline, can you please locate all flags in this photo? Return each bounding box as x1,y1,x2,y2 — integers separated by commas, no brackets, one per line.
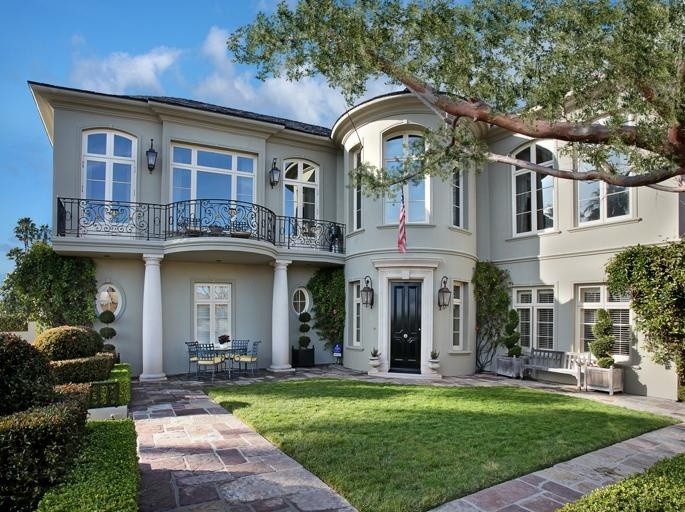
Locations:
398,191,406,253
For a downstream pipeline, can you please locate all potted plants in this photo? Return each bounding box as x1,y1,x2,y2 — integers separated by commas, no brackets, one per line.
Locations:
584,309,625,396
368,348,382,367
218,335,231,348
428,348,441,368
292,312,314,367
492,309,526,379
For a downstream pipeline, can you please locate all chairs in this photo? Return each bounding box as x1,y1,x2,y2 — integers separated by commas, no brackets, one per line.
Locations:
185,340,262,384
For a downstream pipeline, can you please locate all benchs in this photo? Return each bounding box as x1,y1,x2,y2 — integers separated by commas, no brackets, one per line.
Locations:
520,347,591,392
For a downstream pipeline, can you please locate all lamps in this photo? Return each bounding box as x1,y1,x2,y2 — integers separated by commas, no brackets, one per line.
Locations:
146,138,158,173
361,275,374,309
438,276,452,310
269,157,281,188
